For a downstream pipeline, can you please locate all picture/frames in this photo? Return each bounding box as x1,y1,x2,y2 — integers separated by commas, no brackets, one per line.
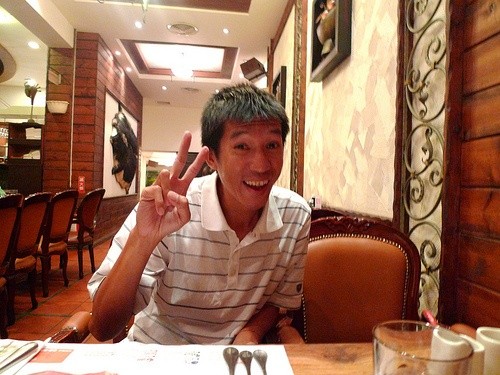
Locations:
309,0,351,84
269,65,287,109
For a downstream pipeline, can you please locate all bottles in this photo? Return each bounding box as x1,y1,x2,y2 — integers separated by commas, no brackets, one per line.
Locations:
78,176,85,199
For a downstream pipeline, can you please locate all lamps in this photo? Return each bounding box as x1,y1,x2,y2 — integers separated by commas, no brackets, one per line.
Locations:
239,59,268,83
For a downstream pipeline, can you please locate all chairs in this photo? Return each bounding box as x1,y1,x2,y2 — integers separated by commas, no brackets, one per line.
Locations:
32,190,79,298
297,215,421,345
0,193,24,339
5,192,51,325
58,188,106,279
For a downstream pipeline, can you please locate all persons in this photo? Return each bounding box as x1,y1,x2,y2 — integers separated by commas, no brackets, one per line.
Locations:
88,81,312,347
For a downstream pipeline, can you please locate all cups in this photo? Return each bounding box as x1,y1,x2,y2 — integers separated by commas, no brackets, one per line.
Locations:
477,327,500,375
429,326,486,375
371,319,473,375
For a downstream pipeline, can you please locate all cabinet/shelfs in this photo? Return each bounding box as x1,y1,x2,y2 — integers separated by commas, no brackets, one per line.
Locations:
0,121,44,193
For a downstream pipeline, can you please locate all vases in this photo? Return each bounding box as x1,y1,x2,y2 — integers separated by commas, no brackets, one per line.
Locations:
46,100,69,114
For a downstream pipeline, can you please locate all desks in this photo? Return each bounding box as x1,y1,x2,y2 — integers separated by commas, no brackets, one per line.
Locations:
0,344,375,375
